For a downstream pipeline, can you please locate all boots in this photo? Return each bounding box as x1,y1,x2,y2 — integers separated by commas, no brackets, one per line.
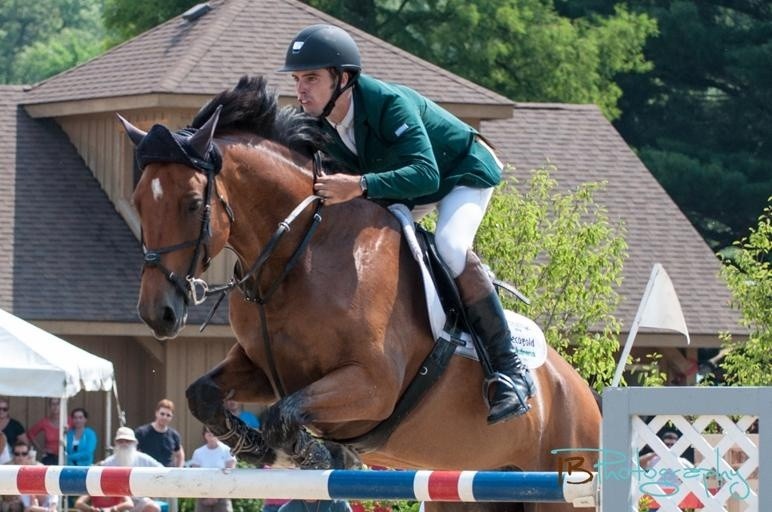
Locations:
453,249,536,425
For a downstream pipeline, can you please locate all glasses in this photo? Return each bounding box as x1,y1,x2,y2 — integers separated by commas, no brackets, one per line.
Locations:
666,443,674,446
13,450,29,456
159,412,171,417
0,407,9,411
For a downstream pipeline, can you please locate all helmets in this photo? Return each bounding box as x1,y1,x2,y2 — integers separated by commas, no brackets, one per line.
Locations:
277,25,362,72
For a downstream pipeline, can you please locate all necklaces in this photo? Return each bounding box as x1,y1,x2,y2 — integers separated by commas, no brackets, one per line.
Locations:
302,500,320,512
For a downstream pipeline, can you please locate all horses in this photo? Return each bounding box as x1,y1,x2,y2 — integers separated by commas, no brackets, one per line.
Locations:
116,74,601,512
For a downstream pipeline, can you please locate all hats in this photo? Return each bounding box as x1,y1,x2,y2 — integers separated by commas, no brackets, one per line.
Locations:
115,427,136,442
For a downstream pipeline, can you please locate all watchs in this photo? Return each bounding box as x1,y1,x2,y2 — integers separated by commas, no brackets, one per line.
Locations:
360,176,367,195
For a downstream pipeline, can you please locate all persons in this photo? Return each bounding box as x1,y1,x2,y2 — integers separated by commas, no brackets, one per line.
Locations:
21,495,59,512
263,464,293,512
279,499,351,512
99,426,165,511
277,24,537,425
75,496,134,512
67,408,97,508
0,432,10,464
134,399,185,512
227,400,259,430
3,441,44,512
27,398,73,466
191,426,238,512
0,398,31,452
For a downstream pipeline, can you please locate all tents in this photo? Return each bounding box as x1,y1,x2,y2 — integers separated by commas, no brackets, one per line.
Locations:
0,307,115,511
